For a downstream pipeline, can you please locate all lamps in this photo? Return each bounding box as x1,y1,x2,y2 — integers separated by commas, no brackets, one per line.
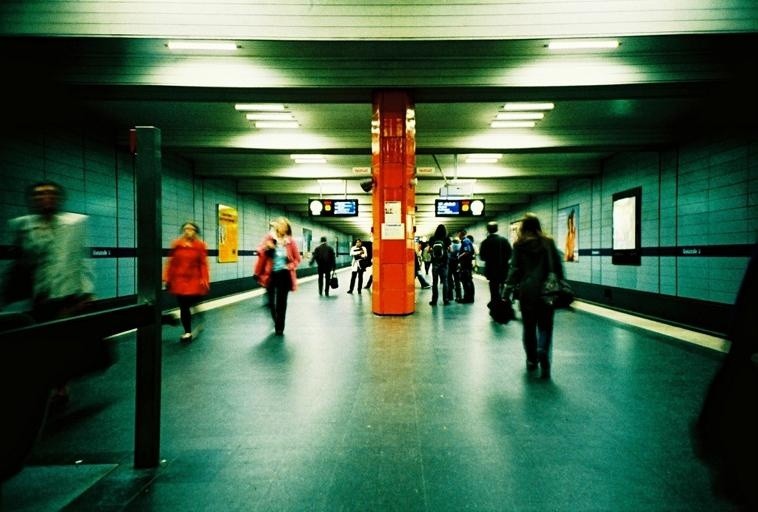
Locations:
290,154,328,164
465,153,503,163
360,180,373,192
543,40,623,50
233,103,304,129
163,41,243,51
488,101,556,128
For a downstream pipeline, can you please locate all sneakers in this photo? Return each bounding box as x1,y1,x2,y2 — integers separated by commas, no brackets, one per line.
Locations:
181,332,192,340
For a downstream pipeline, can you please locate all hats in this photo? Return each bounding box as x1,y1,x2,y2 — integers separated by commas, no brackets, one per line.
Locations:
488,221,497,228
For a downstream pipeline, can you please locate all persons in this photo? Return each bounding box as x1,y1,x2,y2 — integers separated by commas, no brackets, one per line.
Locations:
309,237,336,297
0,180,95,408
348,213,566,379
564,209,576,261
162,222,210,342
253,216,301,337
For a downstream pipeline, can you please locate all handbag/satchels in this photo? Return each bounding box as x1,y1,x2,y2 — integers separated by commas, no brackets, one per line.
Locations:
330,277,338,288
541,272,574,307
359,259,371,268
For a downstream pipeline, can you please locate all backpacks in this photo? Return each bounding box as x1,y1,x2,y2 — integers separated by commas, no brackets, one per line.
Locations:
432,240,446,259
